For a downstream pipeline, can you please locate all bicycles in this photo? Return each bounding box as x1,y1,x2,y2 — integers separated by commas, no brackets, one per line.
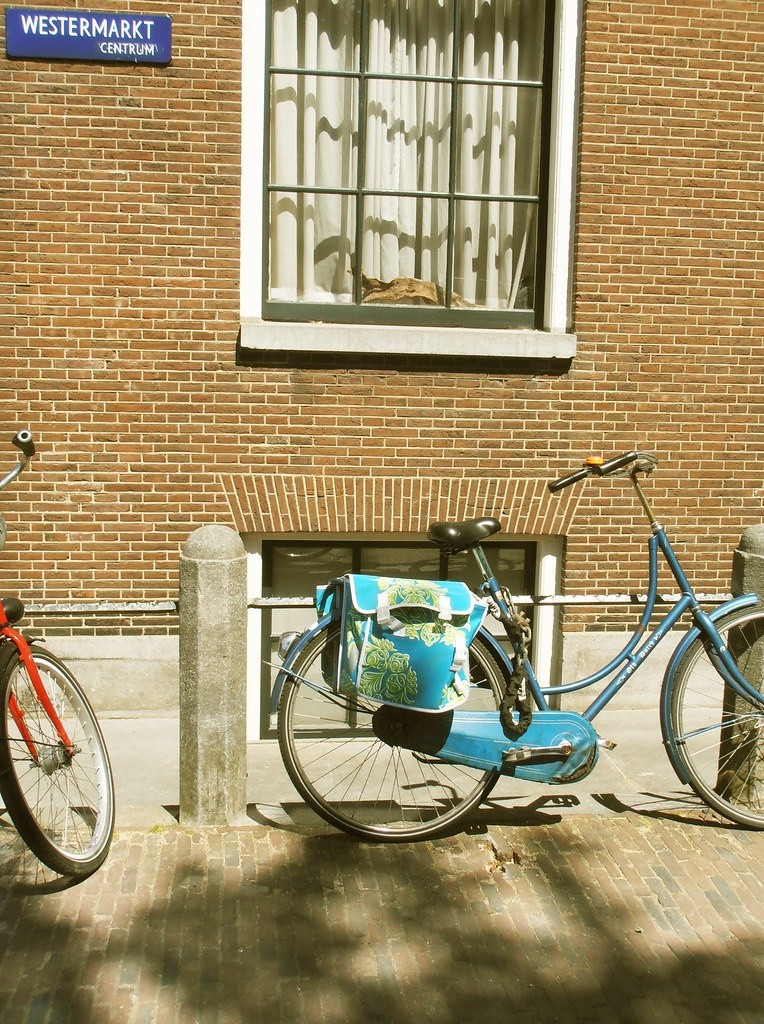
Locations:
268,448,764,842
0,430,119,880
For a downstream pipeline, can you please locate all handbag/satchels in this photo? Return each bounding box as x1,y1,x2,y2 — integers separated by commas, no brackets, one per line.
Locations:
316,574,489,713
